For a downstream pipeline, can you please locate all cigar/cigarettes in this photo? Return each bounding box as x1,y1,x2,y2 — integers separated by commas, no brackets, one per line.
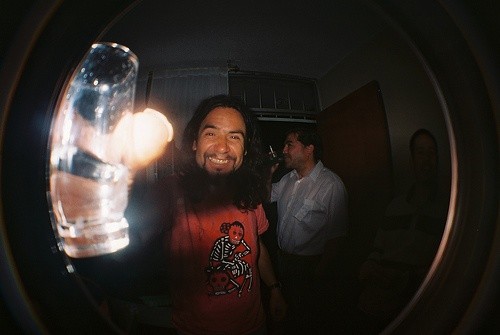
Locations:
269,144,277,159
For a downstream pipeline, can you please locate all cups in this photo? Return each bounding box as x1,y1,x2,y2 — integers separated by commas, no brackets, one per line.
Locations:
49,42,140,258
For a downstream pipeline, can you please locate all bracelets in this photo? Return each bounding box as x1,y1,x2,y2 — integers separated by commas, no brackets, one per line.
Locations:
266,281,284,293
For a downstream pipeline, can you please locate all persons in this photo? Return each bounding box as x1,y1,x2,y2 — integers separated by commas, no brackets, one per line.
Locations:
50,94,288,335
355,128,448,335
265,127,350,335
